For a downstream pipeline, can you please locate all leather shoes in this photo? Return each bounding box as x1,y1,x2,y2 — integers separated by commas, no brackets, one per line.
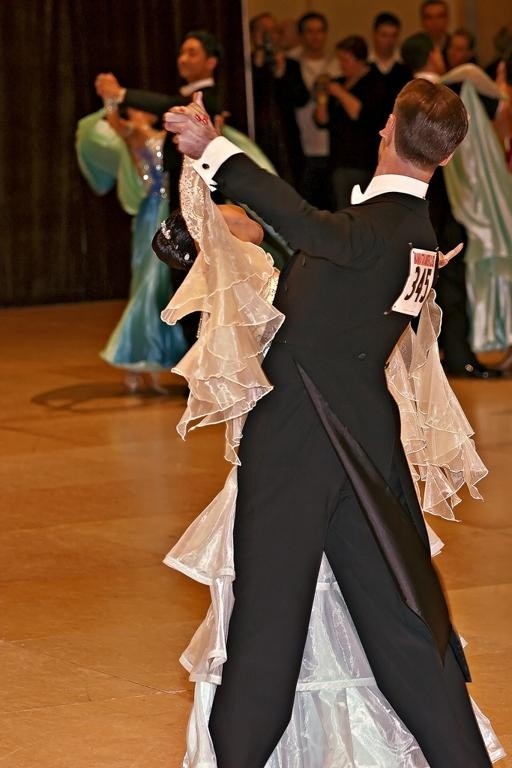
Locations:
445,353,503,378
142,372,168,394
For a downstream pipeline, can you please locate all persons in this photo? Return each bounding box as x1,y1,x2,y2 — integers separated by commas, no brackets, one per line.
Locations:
484,26,511,119
97,32,233,394
311,37,391,210
446,57,511,367
285,9,342,214
404,30,505,378
151,88,509,766
164,76,496,768
366,11,406,113
76,82,280,396
419,2,478,69
248,15,310,195
